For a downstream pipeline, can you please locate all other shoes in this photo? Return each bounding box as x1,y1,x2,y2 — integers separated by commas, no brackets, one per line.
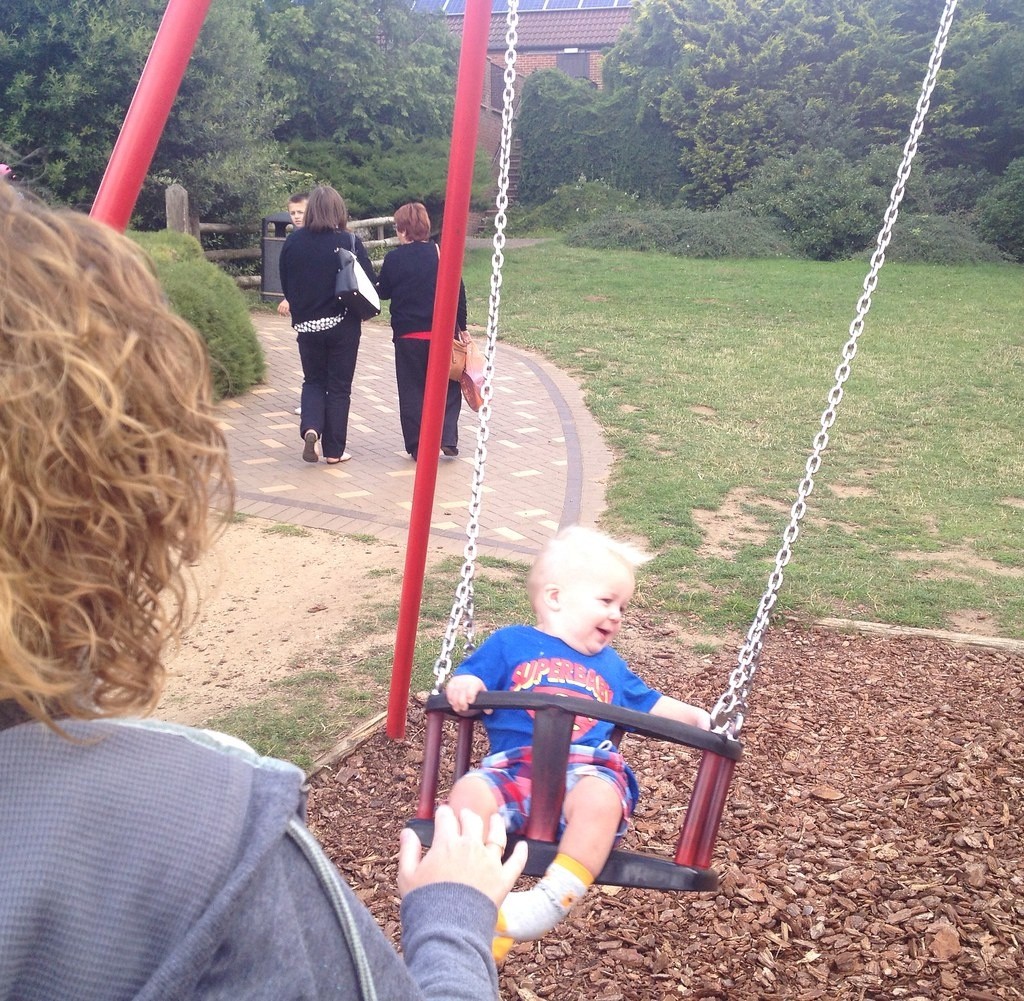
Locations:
441,446,458,456
412,448,417,461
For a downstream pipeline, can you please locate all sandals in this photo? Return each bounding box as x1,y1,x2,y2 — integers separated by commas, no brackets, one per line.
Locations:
327,451,352,464
302,429,319,463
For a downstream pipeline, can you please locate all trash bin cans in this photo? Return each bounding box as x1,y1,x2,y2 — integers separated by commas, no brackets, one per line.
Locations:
260,212,296,305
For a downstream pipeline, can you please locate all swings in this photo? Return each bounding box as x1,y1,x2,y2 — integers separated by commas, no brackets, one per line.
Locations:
412,1,959,891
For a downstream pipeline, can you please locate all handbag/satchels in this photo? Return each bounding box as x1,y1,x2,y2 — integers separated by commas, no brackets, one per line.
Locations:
334,232,382,322
459,339,493,413
448,339,467,381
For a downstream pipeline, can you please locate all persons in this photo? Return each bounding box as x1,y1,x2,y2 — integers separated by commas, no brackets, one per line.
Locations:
434,521,718,961
0,168,528,1001
376,202,472,463
278,185,375,464
276,192,312,415
